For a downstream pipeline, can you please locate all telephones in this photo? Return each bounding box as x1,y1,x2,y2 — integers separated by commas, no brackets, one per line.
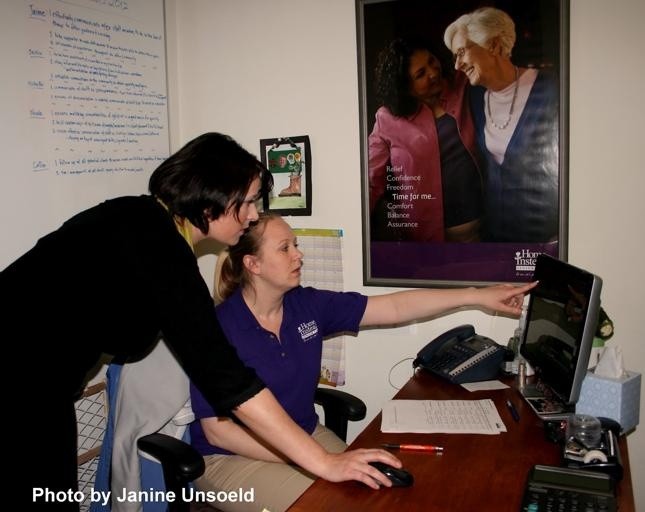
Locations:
413,324,504,385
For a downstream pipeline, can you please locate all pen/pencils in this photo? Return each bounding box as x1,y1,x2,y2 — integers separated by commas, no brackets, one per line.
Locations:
506,400,520,422
381,443,443,452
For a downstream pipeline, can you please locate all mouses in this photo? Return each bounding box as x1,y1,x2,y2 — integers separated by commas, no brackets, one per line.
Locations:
361,461,413,489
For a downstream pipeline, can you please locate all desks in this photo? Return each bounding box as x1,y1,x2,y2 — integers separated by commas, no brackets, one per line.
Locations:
290,369,636,512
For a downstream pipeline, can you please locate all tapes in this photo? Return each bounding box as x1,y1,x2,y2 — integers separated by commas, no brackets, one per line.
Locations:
583,450,608,465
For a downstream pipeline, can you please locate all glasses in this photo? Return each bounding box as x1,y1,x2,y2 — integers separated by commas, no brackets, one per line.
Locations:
454,44,477,61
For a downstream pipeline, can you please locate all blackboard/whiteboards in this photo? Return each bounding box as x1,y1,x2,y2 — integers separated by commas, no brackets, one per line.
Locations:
0,1,176,273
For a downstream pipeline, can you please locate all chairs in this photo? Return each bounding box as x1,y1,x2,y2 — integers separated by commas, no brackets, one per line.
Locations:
88,341,366,512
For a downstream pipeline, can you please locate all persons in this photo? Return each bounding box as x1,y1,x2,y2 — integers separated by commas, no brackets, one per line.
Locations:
441,8,559,243
369,34,491,245
0,136,402,512
188,211,540,512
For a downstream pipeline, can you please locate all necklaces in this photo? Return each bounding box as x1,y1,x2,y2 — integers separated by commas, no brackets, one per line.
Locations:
487,66,520,130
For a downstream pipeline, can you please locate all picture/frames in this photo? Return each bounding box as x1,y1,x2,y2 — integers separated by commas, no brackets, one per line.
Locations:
353,0,570,288
259,136,311,217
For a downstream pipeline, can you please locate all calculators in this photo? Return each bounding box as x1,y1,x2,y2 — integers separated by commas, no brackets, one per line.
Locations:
521,465,617,512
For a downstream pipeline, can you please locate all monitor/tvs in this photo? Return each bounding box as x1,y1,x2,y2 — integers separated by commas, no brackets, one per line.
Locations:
517,253,604,420
